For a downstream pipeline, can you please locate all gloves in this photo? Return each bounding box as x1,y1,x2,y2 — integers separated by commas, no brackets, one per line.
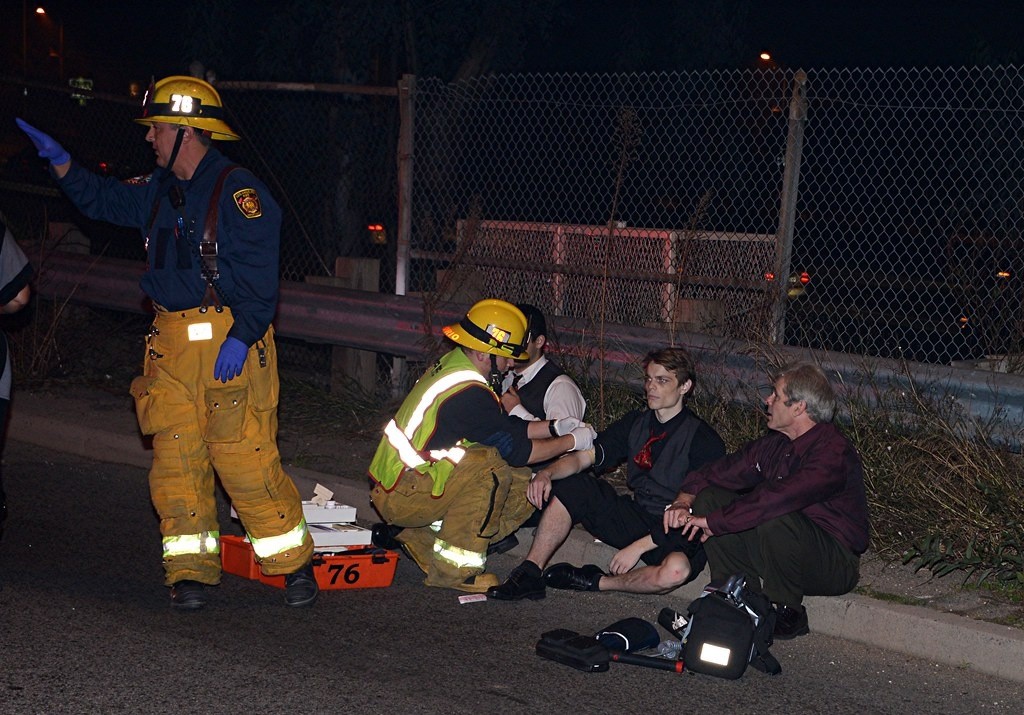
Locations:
214,337,249,382
554,416,585,436
566,427,593,451
16,118,70,165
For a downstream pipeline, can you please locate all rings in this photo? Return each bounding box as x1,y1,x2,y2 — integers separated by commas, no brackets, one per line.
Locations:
685,517,691,522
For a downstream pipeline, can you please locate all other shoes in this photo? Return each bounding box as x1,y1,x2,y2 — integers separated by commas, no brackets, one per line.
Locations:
424,573,498,592
485,532,519,556
404,542,429,574
372,523,405,550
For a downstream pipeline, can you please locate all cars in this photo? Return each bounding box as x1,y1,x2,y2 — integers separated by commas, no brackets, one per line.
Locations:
763,265,813,299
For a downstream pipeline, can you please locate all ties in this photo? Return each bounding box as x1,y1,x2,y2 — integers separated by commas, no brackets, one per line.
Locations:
506,372,523,393
632,431,667,470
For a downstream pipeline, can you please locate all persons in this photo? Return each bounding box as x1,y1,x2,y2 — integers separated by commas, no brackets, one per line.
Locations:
364,299,598,593
663,361,870,641
0,219,33,521
370,302,587,560
484,346,727,601
12,74,320,611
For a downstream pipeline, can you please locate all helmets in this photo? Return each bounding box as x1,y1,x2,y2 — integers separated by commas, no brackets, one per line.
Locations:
442,299,531,360
134,76,241,140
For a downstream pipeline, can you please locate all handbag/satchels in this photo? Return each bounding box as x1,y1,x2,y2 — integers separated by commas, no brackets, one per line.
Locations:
678,591,782,681
740,588,778,657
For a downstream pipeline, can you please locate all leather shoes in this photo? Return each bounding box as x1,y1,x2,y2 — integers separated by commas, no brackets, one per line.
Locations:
774,605,809,639
285,561,319,606
543,562,608,592
171,580,206,608
487,563,546,601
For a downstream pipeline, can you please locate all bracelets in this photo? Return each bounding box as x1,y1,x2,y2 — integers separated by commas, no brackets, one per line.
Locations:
548,419,559,439
